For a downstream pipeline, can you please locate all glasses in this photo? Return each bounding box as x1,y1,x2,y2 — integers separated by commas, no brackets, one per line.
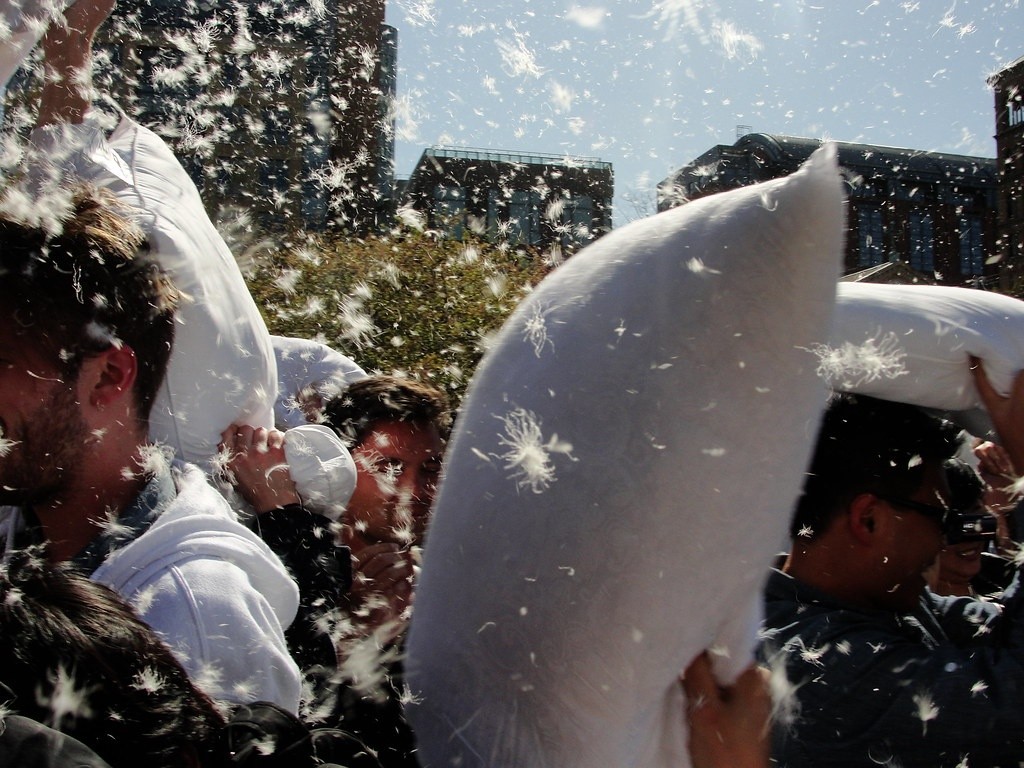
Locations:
886,493,959,535
946,512,997,546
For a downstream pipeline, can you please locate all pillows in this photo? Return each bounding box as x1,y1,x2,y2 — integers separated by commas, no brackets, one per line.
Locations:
1,0,80,102
263,339,370,442
106,112,357,519
402,142,845,768
826,281,1024,448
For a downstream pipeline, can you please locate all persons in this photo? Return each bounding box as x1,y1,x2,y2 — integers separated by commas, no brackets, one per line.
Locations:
0,0,1024,768
